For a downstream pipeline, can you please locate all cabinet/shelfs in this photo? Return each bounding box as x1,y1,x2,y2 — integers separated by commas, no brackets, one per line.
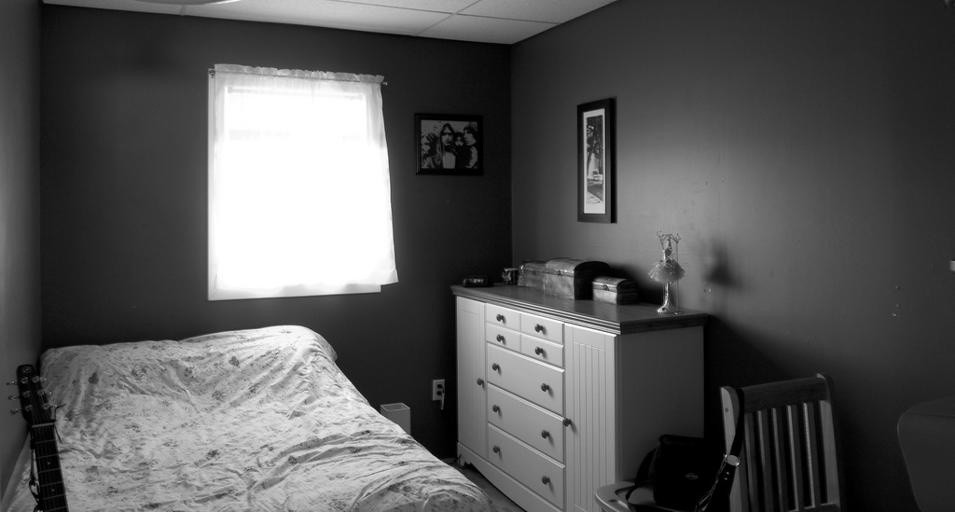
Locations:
564,320,618,512
456,299,489,481
485,298,564,511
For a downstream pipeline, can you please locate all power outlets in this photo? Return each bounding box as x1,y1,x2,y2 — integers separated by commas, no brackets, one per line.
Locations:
432,379,445,401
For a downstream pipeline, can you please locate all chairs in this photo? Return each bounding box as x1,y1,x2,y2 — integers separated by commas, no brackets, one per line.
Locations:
596,374,841,511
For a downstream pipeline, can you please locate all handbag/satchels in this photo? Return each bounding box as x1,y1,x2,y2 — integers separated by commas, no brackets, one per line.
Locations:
625,433,719,511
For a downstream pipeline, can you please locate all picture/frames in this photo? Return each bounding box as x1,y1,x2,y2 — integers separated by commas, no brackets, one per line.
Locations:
414,112,485,177
577,96,617,224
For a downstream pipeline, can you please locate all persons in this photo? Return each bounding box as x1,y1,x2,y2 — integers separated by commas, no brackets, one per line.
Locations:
423,121,464,169
458,121,480,168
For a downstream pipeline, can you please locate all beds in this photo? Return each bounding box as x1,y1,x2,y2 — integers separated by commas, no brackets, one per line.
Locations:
5,322,490,511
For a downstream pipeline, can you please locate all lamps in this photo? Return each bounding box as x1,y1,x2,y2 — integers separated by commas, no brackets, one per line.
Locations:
648,231,684,315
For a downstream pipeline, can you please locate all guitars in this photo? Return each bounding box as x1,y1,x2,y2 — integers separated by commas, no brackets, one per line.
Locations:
4,366,71,512
